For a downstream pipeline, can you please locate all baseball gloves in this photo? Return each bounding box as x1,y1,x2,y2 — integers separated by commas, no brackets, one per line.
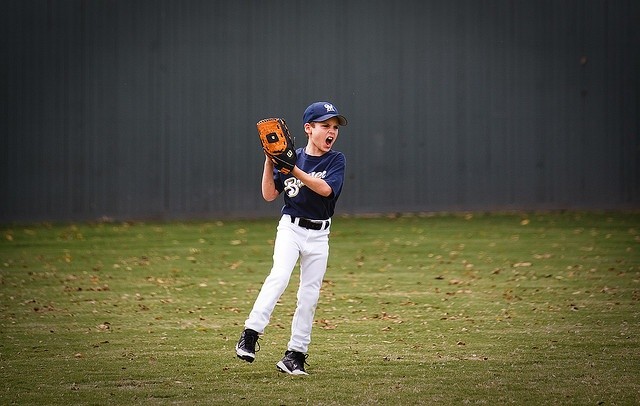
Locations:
256,117,297,174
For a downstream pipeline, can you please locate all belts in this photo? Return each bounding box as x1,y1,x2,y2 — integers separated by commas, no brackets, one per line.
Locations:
289,214,330,231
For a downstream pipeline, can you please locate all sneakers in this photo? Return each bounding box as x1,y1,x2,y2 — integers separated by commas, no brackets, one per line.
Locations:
234,328,261,363
276,351,310,375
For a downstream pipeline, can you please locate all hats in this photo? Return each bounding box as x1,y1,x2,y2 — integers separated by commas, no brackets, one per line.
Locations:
302,101,348,126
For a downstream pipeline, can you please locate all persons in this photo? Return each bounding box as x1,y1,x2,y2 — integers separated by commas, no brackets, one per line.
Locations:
235,100,347,376
